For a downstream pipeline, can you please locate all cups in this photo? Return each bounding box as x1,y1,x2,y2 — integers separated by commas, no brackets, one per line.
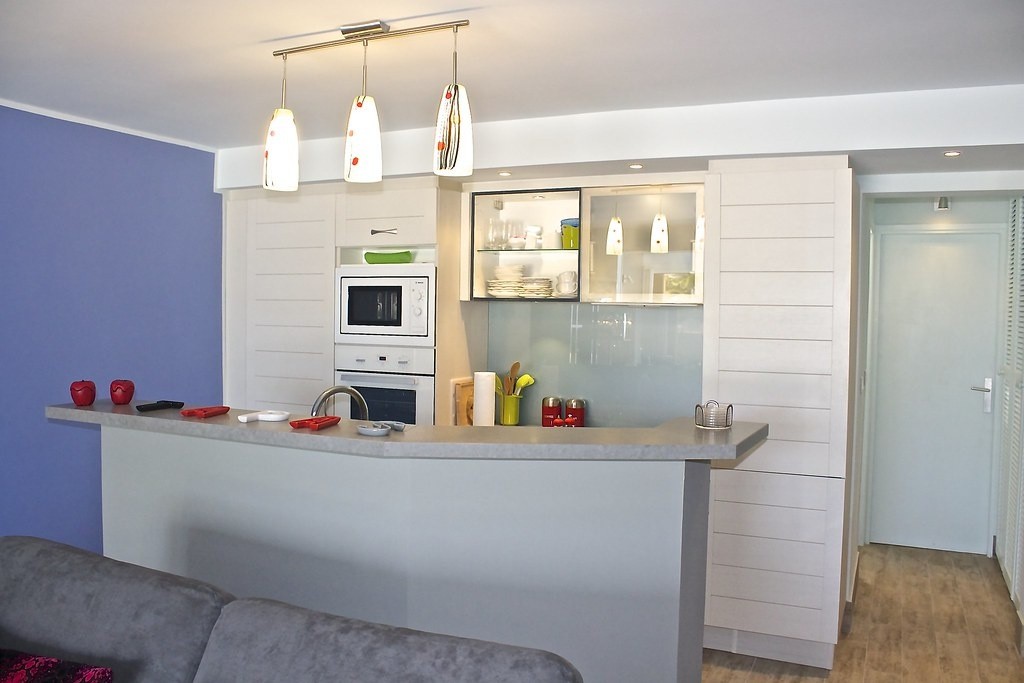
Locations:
484,218,526,250
541,398,586,427
525,232,540,249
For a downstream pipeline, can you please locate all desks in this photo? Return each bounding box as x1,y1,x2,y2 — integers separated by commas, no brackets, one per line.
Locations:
43,393,770,683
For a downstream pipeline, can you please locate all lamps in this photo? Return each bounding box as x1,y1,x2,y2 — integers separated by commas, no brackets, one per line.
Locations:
432,25,475,175
262,54,300,192
344,40,382,183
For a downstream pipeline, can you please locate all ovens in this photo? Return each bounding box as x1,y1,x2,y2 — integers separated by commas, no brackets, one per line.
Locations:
333,264,437,374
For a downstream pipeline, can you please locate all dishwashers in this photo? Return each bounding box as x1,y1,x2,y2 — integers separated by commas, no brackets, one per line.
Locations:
334,371,437,427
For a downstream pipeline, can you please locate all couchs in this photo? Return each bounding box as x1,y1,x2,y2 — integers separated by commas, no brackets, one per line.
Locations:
0,533,588,683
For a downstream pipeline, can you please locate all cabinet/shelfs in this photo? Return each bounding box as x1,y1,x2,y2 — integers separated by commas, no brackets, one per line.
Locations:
459,169,708,308
335,189,437,250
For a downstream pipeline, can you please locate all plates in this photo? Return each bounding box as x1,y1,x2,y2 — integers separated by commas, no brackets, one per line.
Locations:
357,425,391,436
487,266,553,298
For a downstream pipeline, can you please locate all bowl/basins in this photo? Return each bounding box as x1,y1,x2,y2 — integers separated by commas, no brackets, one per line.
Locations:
508,238,525,250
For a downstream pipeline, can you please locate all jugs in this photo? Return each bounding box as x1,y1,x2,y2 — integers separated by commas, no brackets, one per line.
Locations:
556,272,578,295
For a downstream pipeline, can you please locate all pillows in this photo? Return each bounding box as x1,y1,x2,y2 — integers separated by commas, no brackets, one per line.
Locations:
0,648,114,683
